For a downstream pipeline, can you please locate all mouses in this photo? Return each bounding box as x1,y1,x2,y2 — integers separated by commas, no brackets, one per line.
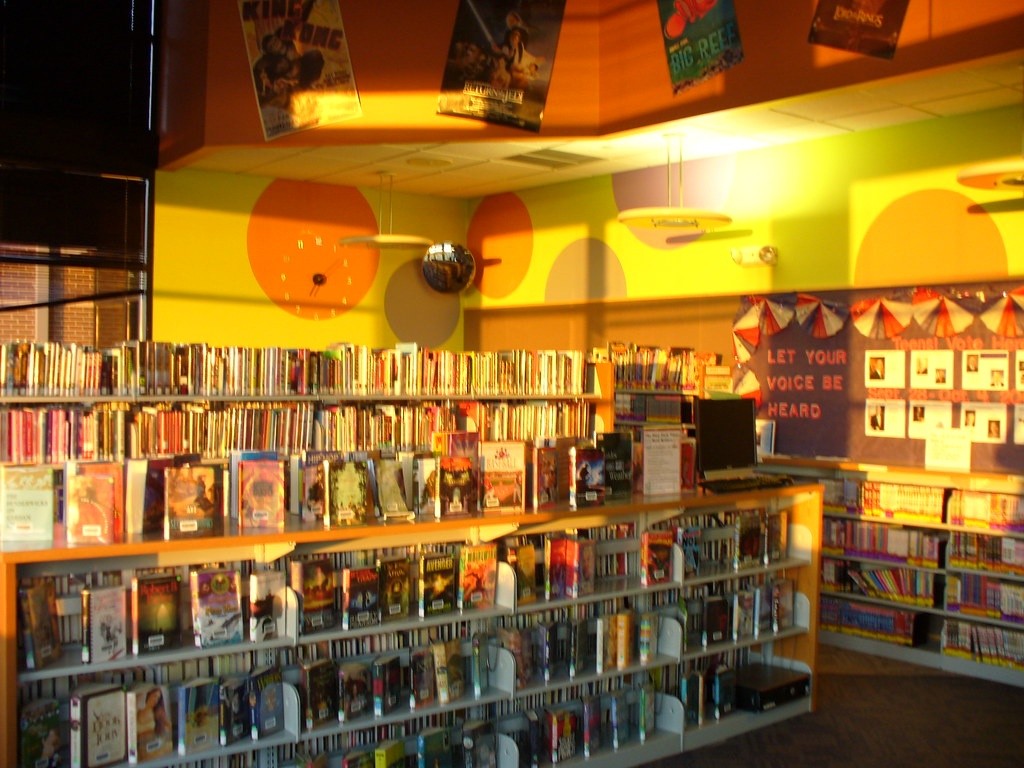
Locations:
779,476,794,487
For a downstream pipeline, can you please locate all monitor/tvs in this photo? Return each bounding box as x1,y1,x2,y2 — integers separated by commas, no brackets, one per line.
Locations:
697,398,759,479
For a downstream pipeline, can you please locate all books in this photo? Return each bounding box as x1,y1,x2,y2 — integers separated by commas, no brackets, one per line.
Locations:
1,340,1024,768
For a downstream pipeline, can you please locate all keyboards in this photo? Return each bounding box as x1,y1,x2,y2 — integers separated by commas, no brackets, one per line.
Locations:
698,474,783,494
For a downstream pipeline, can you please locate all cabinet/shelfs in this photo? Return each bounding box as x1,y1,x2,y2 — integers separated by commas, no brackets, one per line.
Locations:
820,466,1024,692
0,347,821,768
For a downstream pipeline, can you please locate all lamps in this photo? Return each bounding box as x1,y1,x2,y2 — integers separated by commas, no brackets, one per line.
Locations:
616,147,733,228
340,167,434,246
959,96,1024,190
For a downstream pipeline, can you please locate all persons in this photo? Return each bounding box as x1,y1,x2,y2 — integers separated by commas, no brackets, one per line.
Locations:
917,360,927,375
870,358,885,380
991,371,1004,388
870,406,884,432
987,422,1000,438
257,13,544,118
914,406,925,422
965,411,975,427
967,357,978,371
935,369,946,384
